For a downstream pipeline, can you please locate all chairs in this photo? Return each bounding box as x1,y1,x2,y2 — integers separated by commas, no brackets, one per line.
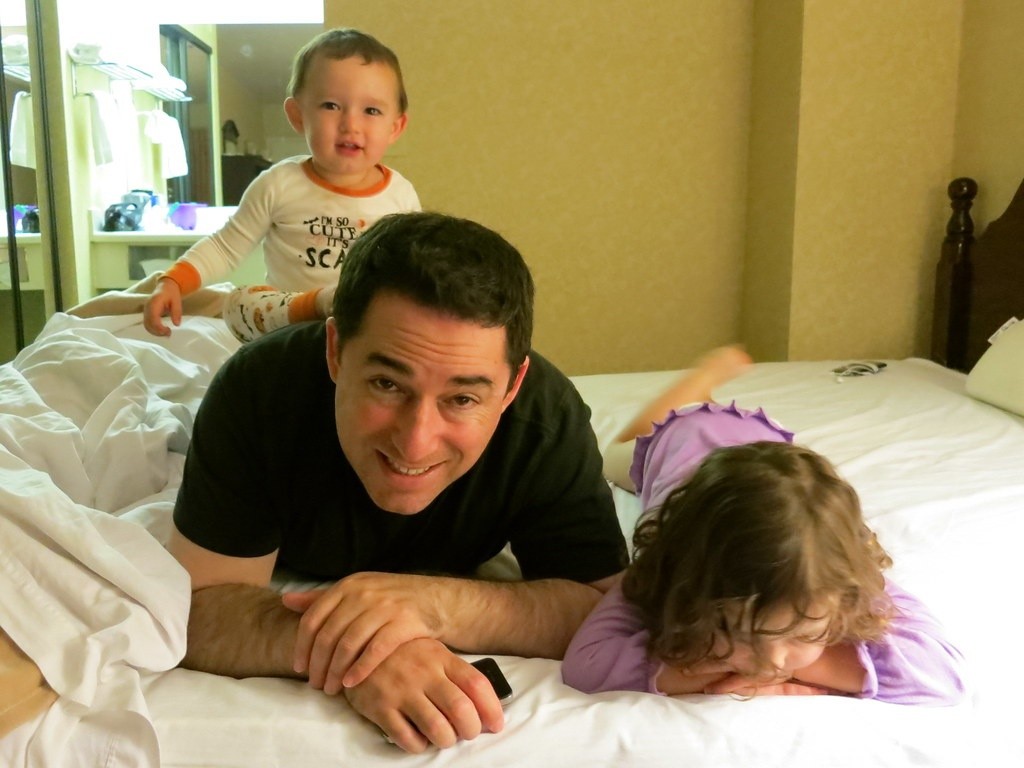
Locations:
1,178,1023,768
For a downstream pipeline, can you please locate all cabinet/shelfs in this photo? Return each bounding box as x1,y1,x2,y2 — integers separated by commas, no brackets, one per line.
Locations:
88,210,207,297
222,155,262,206
0,232,43,290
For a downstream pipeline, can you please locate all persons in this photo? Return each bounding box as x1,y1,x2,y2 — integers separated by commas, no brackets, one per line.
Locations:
144,27,425,350
166,213,631,754
561,345,975,704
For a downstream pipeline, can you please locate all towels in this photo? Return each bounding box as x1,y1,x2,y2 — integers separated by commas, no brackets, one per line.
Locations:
144,110,188,179
68,35,135,76
88,90,142,166
9,90,36,171
1,34,29,78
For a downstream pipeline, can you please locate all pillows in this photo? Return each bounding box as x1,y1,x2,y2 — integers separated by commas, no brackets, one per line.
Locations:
964,319,1023,417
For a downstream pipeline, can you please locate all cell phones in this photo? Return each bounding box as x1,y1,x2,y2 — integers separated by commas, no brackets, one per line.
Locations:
371,657,514,748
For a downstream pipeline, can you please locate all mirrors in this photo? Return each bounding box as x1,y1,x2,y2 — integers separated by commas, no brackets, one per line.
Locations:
160,23,323,207
1,0,63,366
